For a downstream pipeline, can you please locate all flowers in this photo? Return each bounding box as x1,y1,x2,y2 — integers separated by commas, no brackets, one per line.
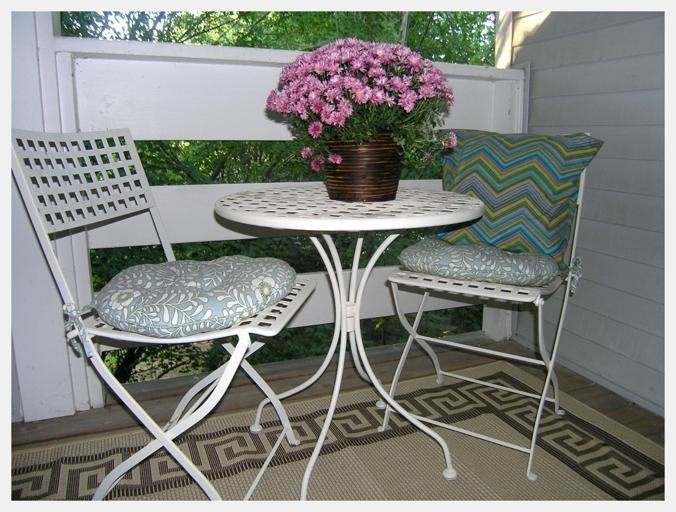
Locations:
266,37,458,173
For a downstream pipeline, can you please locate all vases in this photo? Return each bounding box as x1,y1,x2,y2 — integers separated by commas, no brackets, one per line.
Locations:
304,132,407,202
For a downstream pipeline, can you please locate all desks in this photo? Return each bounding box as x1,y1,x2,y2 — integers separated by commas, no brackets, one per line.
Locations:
210,181,490,501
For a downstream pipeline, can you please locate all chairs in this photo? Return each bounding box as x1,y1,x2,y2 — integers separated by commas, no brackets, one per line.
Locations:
376,129,602,486
12,127,303,501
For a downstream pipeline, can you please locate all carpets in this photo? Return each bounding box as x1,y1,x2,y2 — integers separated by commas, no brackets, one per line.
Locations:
12,361,665,500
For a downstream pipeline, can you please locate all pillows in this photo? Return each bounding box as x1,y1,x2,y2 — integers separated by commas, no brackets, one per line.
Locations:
393,236,563,290
91,251,299,338
